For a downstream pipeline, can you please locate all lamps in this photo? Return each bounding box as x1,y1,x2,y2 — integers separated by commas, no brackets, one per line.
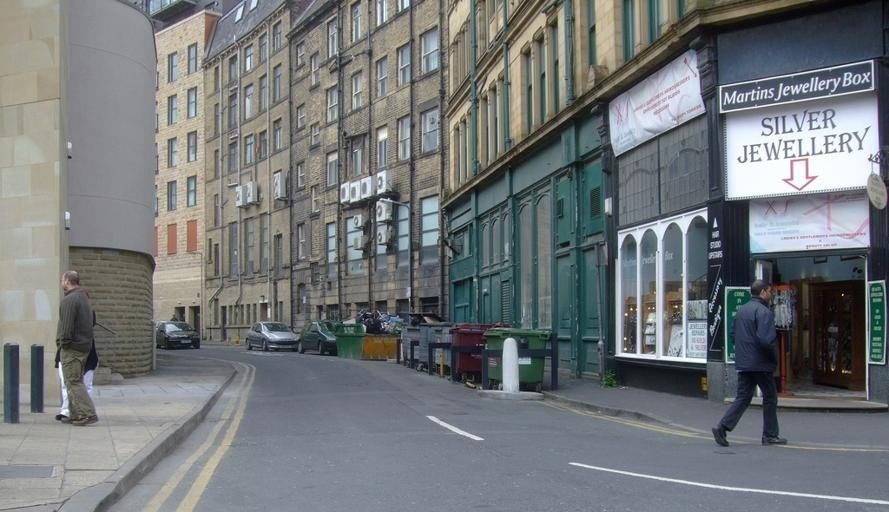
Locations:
379,197,410,209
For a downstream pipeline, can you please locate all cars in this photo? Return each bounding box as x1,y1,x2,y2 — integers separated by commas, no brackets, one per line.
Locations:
157,322,201,349
244,321,300,352
156,320,174,330
296,319,338,356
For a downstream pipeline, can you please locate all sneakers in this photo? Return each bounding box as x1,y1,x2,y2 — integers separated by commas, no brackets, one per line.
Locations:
761,434,787,446
55,410,101,427
711,424,730,447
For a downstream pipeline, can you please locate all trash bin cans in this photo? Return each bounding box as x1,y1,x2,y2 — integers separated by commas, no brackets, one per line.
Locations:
402,311,553,393
333,323,366,362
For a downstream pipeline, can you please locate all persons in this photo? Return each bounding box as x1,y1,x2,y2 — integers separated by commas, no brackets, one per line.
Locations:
171,314,178,321
711,279,788,446
53,270,98,426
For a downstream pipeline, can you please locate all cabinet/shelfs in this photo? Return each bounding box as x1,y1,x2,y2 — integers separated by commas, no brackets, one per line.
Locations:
788,274,824,380
810,279,866,391
624,287,696,356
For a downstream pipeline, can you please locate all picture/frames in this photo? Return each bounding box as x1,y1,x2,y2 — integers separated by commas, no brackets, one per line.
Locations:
813,256,827,264
839,255,865,262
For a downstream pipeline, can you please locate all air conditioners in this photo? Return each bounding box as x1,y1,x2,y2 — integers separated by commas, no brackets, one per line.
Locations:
353,214,368,229
353,236,370,250
377,230,392,246
234,171,287,210
339,169,393,205
374,198,392,225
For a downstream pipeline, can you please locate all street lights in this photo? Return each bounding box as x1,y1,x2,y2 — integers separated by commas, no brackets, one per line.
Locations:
380,195,415,327
